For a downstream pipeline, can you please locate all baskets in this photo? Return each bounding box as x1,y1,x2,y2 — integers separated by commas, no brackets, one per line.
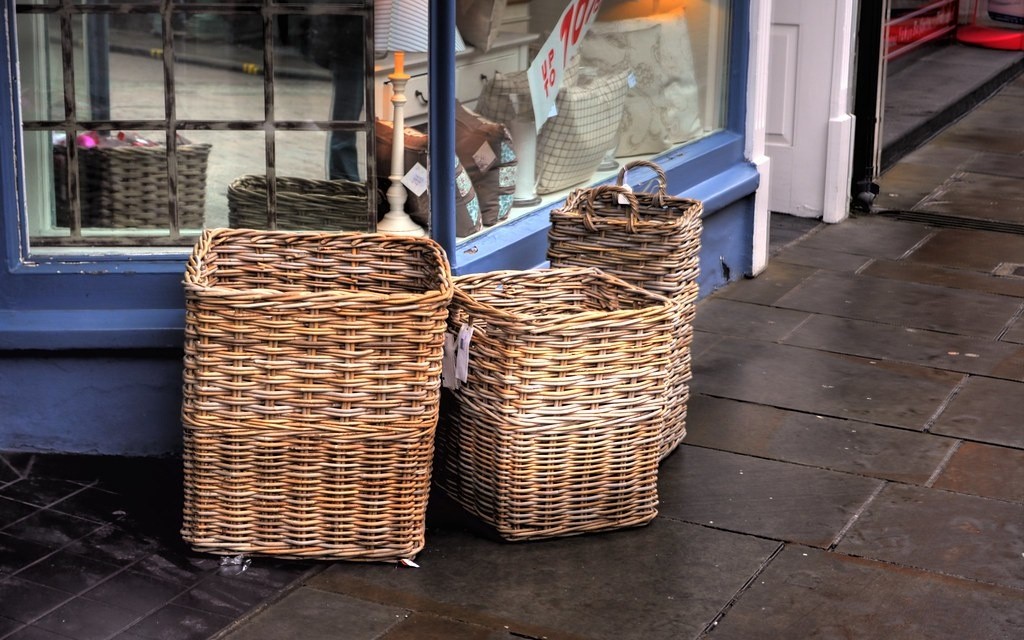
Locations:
180,227,453,565
444,266,681,546
49,132,214,236
551,159,704,463
226,169,376,232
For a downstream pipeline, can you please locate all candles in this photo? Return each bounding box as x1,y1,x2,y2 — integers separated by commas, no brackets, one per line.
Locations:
509,118,537,199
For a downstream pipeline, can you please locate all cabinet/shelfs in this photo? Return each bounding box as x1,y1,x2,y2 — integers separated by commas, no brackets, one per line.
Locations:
374,0,540,128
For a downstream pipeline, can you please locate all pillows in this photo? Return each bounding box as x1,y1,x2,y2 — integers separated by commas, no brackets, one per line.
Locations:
474,51,581,127
454,98,518,228
534,65,633,196
376,120,483,237
542,23,674,159
591,5,703,143
456,0,508,53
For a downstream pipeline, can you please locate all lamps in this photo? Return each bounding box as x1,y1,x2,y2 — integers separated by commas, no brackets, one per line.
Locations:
374,0,466,237
